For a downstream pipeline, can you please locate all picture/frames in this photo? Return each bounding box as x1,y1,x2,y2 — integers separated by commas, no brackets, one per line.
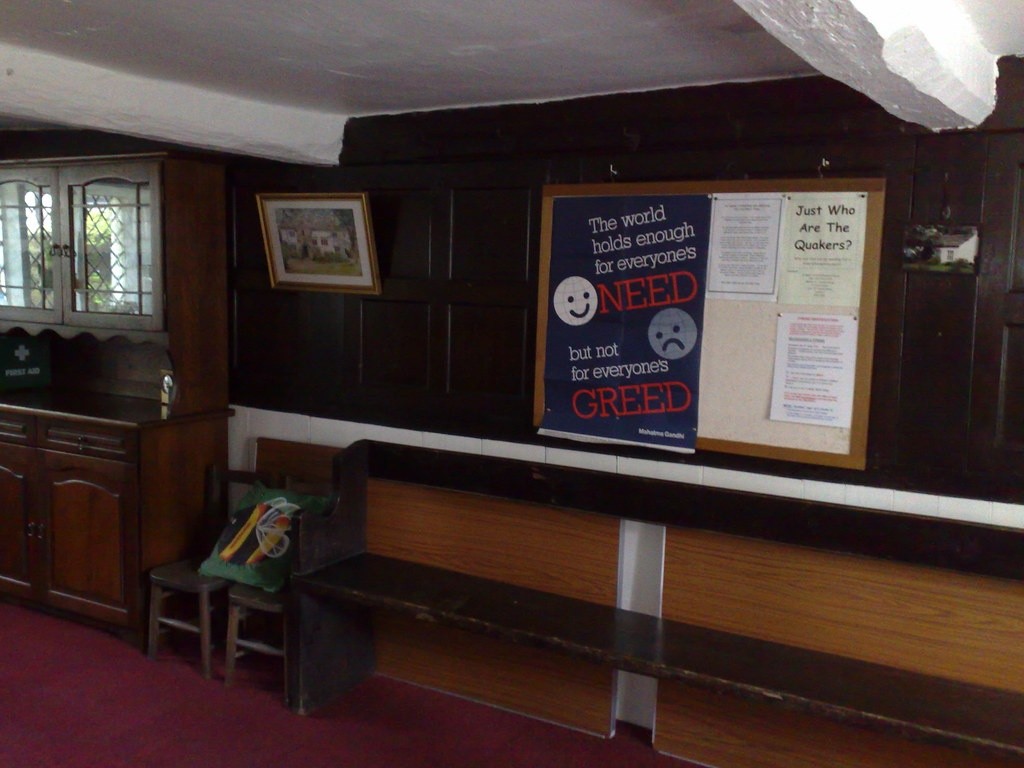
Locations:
255,192,382,296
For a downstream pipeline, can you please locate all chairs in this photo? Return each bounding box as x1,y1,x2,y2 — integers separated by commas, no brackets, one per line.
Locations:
148,462,295,680
223,583,297,706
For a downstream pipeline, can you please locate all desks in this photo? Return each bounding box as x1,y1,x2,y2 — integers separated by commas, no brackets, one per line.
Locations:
0,389,237,652
